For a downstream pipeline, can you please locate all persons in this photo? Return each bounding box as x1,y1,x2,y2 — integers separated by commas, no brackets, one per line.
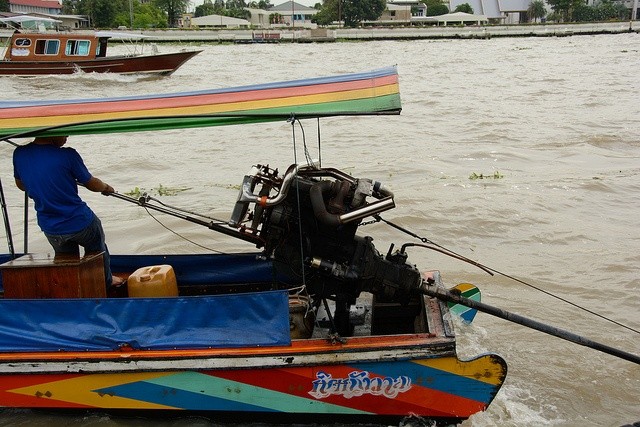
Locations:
13,135,128,291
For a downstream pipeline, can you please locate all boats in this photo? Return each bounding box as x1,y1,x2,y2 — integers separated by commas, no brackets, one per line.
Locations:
0,30,204,81
0,63,639,427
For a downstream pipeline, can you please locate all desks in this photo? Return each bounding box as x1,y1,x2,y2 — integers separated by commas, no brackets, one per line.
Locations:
0,250,108,299
